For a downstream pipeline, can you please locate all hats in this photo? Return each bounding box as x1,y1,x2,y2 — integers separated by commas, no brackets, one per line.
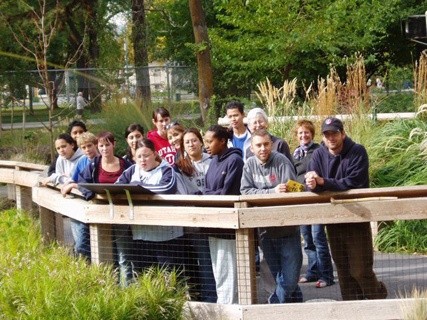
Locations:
321,117,343,133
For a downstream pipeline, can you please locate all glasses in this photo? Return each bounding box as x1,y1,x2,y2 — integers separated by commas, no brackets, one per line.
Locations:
165,121,180,131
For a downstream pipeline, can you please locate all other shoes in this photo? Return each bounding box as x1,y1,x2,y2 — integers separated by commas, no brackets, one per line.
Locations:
299,277,308,283
315,279,327,287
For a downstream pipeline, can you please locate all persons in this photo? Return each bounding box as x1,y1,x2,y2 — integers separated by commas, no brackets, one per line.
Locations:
242,108,297,175
203,125,243,304
79,133,133,289
226,100,251,150
113,139,188,281
76,92,88,123
48,121,89,177
42,134,85,253
291,120,334,288
304,118,387,300
62,131,101,264
119,123,147,163
165,122,188,168
239,131,305,303
146,107,177,165
180,129,217,303
